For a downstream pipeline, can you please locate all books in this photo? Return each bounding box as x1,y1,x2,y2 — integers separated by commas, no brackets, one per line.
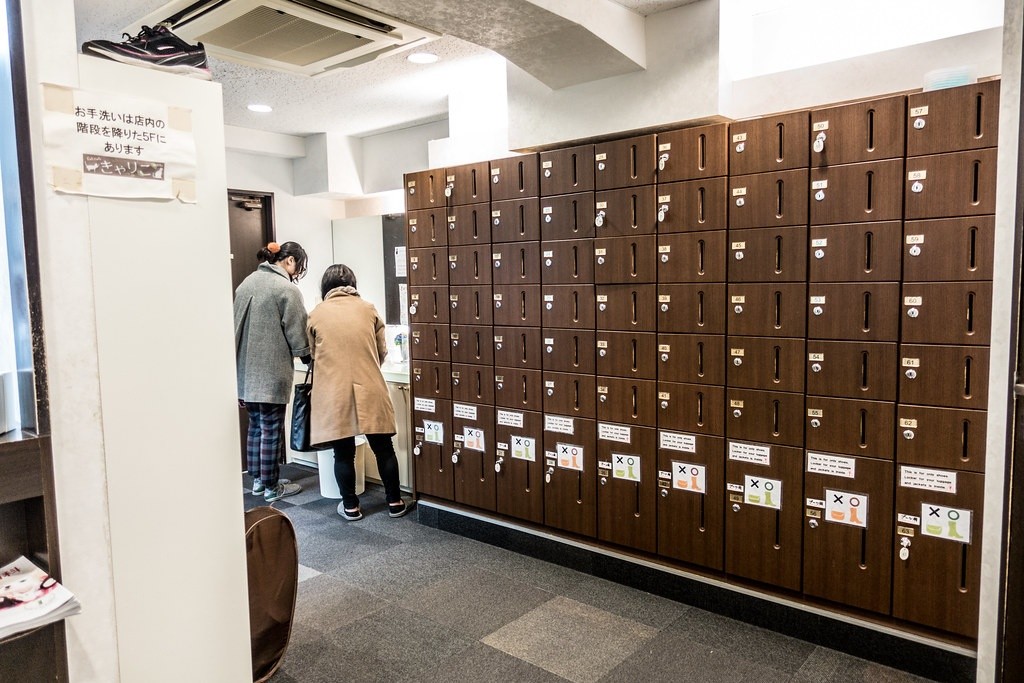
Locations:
0,556,82,638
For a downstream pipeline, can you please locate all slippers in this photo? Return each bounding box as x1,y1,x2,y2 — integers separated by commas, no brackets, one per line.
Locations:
388,495,415,517
263,482,302,502
337,500,363,521
252,477,292,496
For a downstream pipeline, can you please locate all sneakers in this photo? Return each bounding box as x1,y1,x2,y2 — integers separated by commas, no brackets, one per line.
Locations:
80,21,213,81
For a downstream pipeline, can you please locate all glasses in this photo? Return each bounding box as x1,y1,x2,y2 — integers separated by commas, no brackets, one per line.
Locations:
298,263,306,274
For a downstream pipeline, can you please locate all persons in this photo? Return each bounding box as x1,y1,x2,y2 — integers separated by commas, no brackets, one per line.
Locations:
0,568,58,610
305,264,413,520
234,241,312,502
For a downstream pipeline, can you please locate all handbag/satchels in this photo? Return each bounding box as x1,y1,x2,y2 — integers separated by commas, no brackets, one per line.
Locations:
289,358,333,452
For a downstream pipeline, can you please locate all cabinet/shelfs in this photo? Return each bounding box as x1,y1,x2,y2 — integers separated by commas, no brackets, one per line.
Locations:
283,363,412,493
402,80,1024,683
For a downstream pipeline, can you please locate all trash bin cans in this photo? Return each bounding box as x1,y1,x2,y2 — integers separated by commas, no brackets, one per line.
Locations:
316,438,366,499
